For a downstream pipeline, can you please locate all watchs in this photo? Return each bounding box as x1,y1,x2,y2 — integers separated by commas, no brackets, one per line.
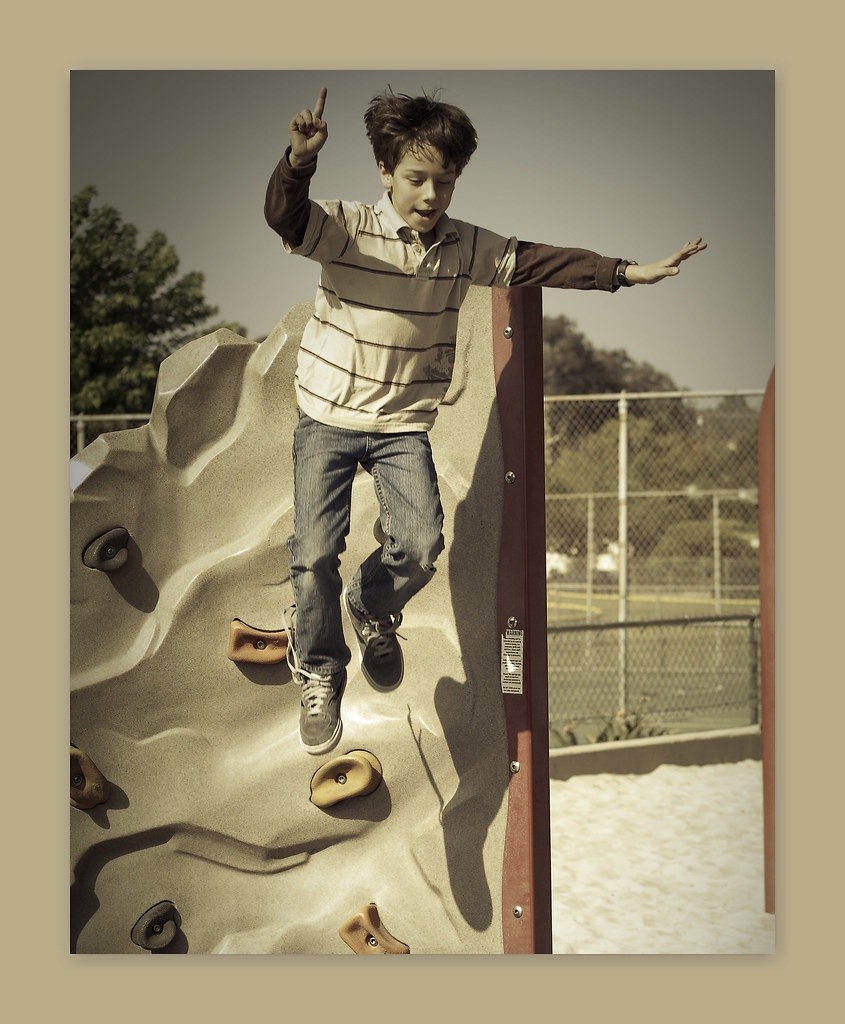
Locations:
618,260,638,289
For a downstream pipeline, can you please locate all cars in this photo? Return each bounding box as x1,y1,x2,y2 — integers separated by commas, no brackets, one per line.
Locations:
546,533,636,580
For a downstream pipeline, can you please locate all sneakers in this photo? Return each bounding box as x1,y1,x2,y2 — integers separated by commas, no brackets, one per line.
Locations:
342,589,405,692
299,668,349,757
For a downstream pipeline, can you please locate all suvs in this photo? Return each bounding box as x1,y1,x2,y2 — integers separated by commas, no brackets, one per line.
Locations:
652,518,761,589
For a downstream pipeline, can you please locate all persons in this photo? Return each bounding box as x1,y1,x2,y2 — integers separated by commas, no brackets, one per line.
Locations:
264,82,707,755
